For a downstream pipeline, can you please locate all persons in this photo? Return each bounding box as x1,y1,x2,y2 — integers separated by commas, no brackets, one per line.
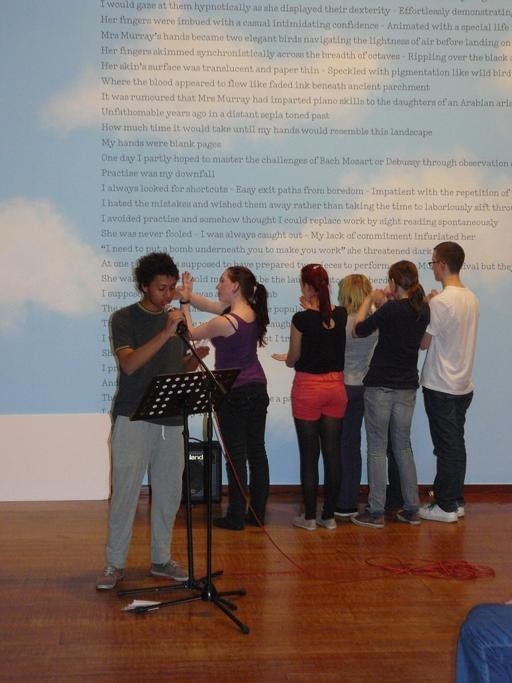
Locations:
95,253,189,591
454,601,512,683
175,265,271,530
351,259,431,526
320,273,379,517
418,240,481,522
271,263,347,532
364,283,427,512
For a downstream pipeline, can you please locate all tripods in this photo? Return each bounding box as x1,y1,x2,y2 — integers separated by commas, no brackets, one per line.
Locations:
116,415,242,611
131,337,252,635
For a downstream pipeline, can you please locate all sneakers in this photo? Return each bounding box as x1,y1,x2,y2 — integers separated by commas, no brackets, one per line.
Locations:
151,559,188,581
291,513,316,530
396,509,421,524
96,566,125,589
350,513,384,528
315,516,336,529
424,503,465,518
321,505,354,516
418,504,457,523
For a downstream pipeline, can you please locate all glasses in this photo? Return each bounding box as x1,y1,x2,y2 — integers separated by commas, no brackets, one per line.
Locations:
427,261,439,269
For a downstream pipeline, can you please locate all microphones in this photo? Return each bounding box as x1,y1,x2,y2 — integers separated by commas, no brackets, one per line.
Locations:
161,303,191,337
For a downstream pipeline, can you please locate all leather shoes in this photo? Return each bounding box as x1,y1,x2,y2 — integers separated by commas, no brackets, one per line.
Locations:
244,513,263,526
214,518,242,530
